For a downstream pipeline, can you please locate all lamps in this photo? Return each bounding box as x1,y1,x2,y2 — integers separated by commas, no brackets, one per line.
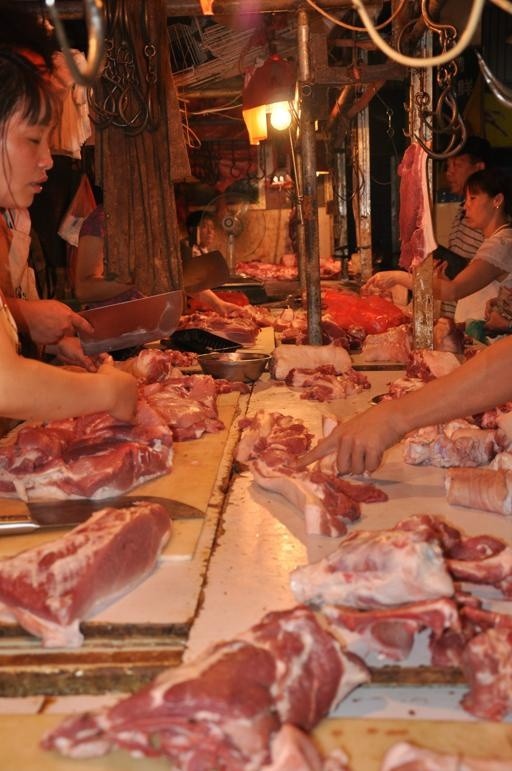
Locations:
243,55,297,131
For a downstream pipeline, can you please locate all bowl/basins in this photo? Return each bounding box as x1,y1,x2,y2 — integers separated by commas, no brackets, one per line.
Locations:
197,352,271,383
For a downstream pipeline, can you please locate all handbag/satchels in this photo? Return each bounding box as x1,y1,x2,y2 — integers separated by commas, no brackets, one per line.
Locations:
431,246,467,279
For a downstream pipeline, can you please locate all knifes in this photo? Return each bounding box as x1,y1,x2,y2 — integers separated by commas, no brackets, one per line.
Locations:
74,290,184,356
182,252,232,298
0,495,207,533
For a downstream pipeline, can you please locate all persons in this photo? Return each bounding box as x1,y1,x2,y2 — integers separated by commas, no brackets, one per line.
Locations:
180,210,216,260
286,333,512,476
437,135,497,319
73,205,245,363
364,168,512,326
482,287,512,330
1,207,117,373
0,47,140,438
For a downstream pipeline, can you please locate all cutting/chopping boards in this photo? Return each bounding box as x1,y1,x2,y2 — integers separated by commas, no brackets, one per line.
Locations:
0,381,251,636
1,709,512,771
183,372,512,720
345,344,410,369
